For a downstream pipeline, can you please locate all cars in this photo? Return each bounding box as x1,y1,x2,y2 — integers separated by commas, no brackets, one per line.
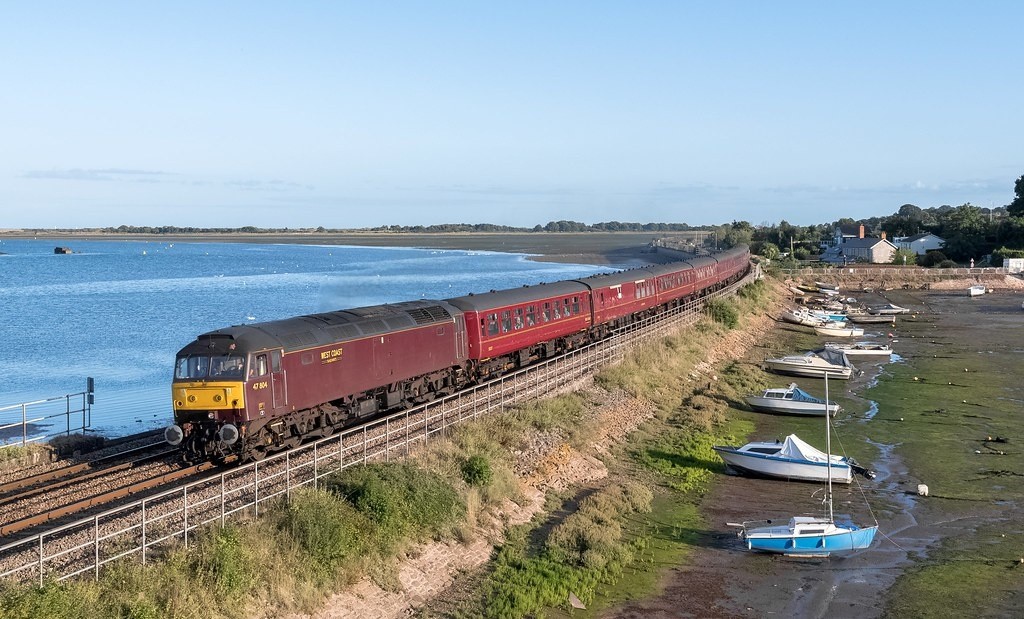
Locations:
779,253,788,258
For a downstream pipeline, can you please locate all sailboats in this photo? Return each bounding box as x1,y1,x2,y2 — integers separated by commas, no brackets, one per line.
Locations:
726,369,879,559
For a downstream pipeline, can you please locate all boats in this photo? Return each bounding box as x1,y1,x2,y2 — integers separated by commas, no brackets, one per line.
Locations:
741,383,840,419
815,282,839,290
798,285,820,292
786,286,806,296
711,433,876,485
817,289,840,295
824,342,893,356
813,326,864,336
782,296,910,327
758,350,864,380
985,289,993,293
850,287,873,293
883,287,894,291
966,285,986,297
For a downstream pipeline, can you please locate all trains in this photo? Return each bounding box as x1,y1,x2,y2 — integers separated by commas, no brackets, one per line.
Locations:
165,238,751,459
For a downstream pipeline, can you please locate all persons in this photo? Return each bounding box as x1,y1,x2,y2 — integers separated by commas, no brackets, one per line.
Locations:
240,362,254,377
502,306,570,333
